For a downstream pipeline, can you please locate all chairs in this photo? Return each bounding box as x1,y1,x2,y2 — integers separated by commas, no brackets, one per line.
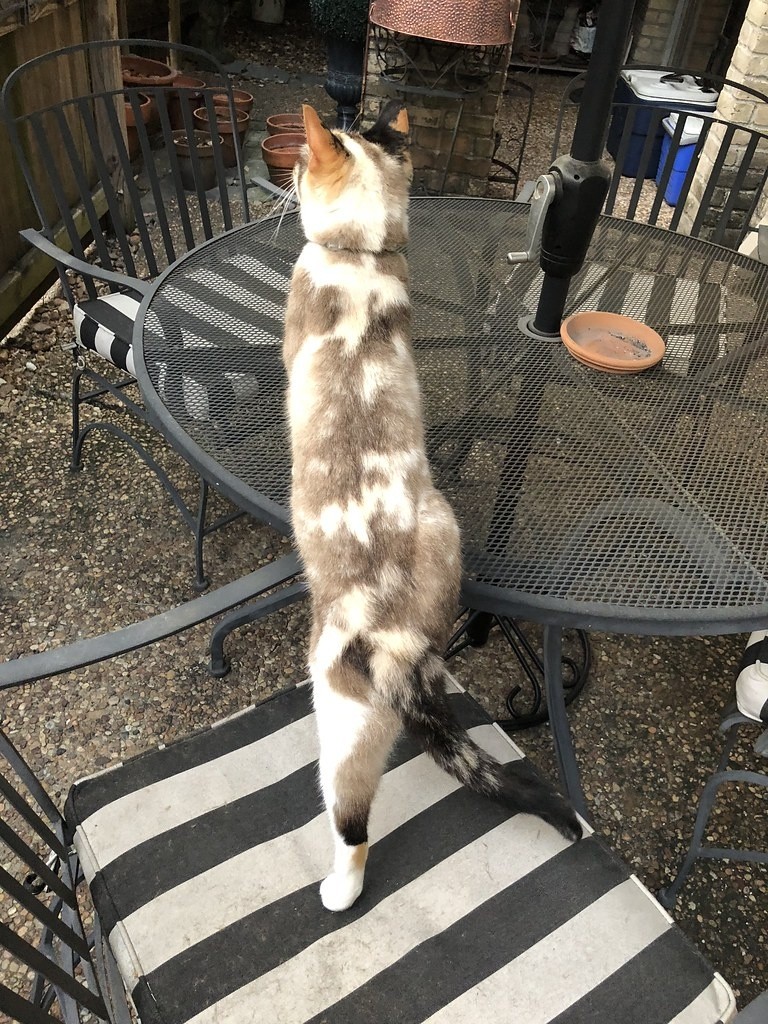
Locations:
428,63,768,511
0,38,303,592
656,627,768,910
0,551,768,1024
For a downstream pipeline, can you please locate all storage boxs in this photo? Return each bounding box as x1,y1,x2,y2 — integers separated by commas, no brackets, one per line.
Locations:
605,67,720,179
651,109,714,208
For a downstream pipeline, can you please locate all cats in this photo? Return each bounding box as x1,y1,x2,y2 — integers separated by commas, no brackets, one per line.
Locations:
279,101,464,911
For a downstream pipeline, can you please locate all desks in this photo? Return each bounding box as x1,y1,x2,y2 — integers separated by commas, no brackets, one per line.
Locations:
132,194,767,727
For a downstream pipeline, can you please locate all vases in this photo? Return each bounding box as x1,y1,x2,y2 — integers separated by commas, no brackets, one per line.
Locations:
118,52,307,193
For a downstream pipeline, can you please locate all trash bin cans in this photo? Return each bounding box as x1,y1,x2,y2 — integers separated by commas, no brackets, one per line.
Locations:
606,70,718,179
656,110,713,207
